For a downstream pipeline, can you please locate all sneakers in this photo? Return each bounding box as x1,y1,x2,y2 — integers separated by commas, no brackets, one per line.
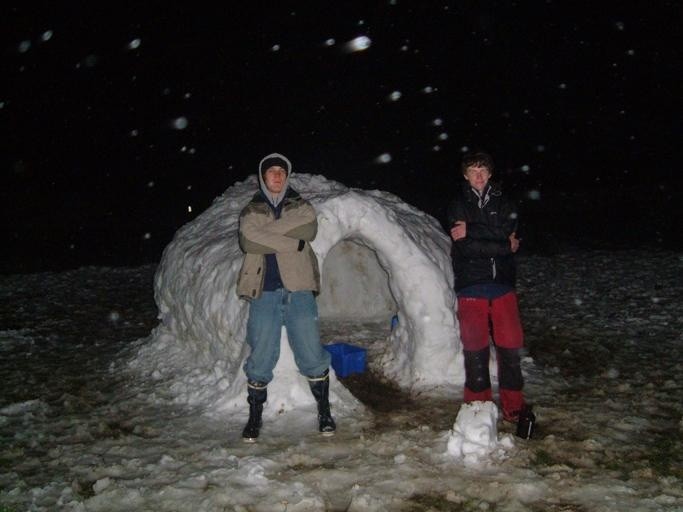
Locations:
504,408,535,426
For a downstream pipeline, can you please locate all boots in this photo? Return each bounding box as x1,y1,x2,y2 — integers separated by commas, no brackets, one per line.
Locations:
307,368,336,436
242,379,267,444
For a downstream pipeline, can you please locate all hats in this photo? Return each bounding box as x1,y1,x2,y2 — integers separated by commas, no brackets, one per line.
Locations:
262,158,288,175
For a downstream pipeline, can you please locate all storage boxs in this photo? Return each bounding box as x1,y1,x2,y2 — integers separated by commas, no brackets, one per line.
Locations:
322,341,367,380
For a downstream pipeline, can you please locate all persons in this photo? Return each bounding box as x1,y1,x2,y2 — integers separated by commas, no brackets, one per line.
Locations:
447,150,524,424
236,152,335,443
